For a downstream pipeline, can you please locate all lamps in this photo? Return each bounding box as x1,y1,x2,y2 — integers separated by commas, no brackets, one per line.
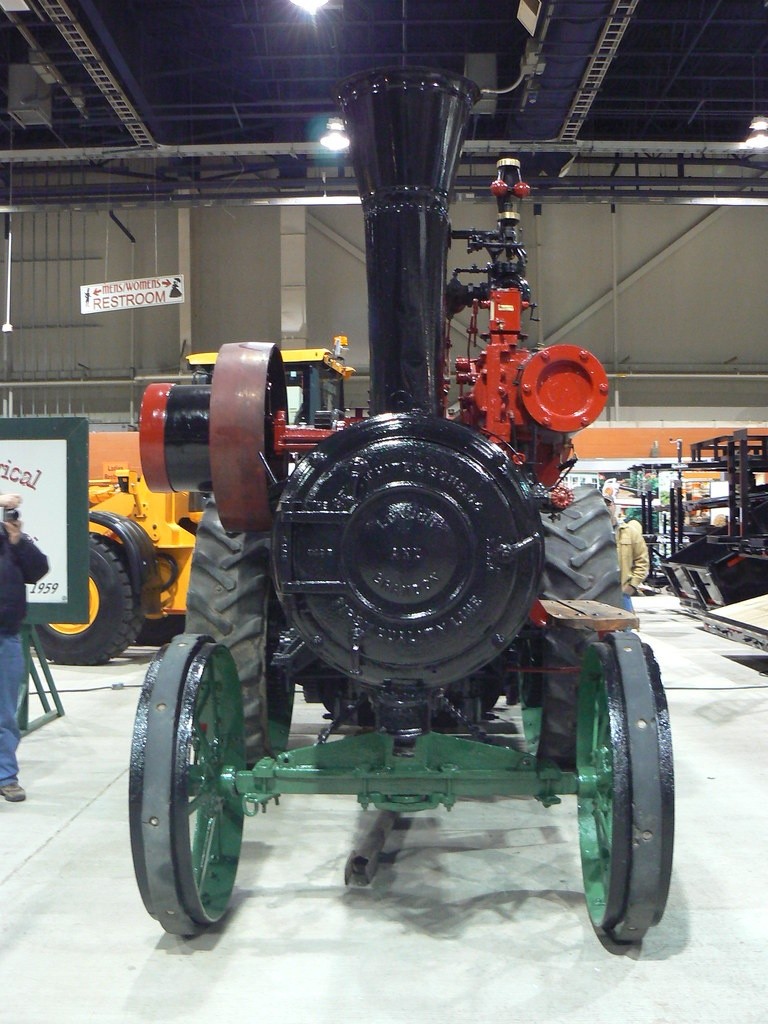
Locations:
320,117,350,150
746,116,768,151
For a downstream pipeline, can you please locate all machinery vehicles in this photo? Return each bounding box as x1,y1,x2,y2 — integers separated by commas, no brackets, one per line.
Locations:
30,335,355,667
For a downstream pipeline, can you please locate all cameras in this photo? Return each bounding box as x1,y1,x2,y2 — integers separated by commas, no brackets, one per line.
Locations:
3,509,19,522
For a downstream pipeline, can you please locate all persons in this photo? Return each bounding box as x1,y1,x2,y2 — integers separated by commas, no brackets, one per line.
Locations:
602,494,650,631
0,495,50,803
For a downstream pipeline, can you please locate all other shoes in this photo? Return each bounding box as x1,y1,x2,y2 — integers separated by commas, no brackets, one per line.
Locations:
0,782,25,802
